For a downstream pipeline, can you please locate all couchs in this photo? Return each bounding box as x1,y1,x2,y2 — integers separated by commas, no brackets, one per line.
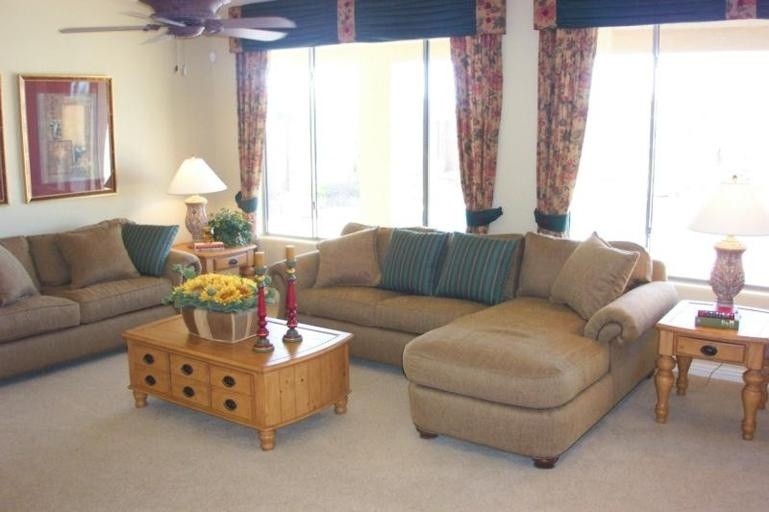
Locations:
269,224,677,470
0,218,202,382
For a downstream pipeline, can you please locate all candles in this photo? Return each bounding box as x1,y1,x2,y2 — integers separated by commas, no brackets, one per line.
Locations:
252,251,274,276
282,244,303,268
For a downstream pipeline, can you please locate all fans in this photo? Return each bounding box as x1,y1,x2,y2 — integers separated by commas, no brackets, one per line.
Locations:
60,0,297,51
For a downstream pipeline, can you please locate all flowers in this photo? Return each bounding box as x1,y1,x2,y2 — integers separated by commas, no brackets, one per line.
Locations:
159,272,279,315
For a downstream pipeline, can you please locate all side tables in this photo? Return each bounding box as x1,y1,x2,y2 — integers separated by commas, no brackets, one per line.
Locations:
654,299,769,441
170,242,258,277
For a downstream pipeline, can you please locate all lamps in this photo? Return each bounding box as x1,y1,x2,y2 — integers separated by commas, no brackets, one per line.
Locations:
666,174,768,312
165,155,228,248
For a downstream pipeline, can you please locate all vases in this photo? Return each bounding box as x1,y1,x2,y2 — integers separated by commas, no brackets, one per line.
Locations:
181,304,259,344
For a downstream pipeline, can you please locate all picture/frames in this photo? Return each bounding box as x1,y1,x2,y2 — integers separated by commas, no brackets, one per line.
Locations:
19,73,119,204
0,73,8,205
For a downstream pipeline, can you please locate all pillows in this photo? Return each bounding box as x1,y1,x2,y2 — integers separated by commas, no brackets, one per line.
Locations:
120,223,179,277
57,220,141,290
312,226,382,290
514,231,583,297
434,231,518,305
381,227,451,297
548,230,640,322
0,242,39,306
